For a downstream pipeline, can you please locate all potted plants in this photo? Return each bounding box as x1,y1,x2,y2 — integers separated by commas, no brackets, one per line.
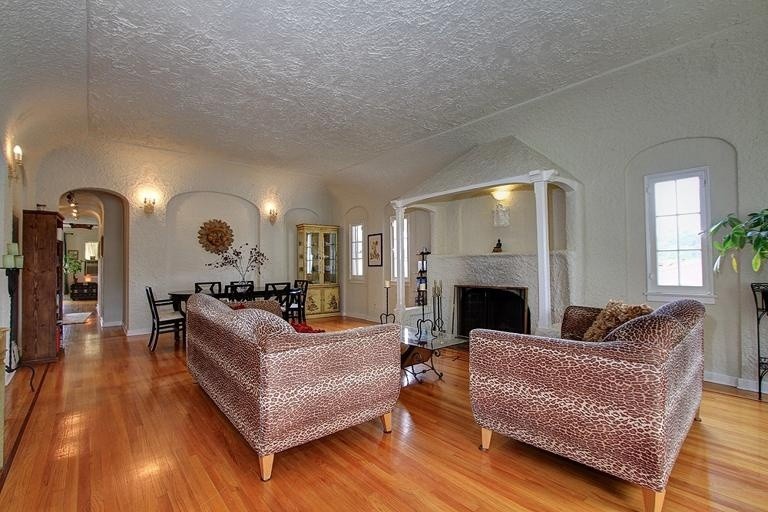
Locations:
205,243,269,292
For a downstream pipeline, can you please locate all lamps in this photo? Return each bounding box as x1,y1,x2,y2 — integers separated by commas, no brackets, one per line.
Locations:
490,190,510,200
0,242,35,392
13,144,27,186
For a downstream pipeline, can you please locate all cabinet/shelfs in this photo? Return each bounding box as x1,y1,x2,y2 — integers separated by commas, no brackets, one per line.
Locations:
24,210,63,362
296,224,342,317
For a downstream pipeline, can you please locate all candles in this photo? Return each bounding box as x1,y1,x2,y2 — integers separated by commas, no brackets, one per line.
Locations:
420,283,426,290
384,281,391,288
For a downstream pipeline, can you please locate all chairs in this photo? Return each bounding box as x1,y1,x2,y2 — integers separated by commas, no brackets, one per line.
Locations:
145,280,309,352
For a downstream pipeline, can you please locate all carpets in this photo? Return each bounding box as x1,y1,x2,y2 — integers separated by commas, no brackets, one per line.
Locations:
62,311,93,325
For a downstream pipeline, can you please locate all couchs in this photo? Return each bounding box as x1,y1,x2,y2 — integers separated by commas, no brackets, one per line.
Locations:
187,292,402,480
468,299,704,512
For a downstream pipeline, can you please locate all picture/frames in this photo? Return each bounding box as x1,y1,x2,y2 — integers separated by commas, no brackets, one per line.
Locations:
367,233,383,266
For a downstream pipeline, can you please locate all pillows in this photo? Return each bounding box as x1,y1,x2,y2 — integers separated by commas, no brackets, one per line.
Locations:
583,299,654,341
234,309,297,334
